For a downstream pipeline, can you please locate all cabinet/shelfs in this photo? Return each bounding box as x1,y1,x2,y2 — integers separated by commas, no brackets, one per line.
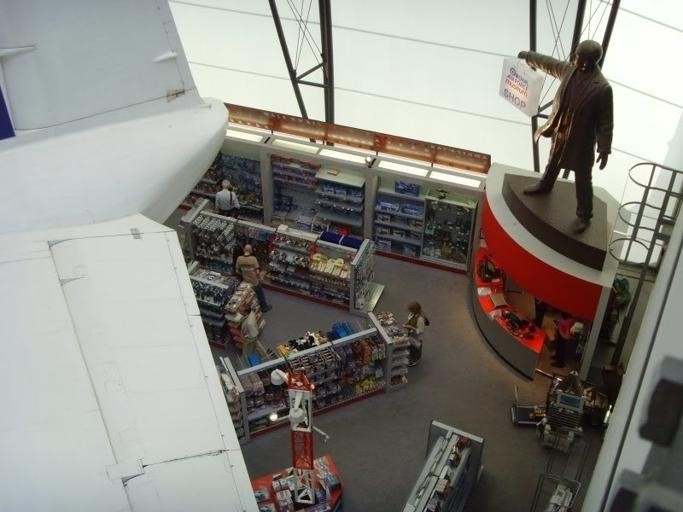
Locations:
180,198,385,315
186,260,264,352
218,311,409,444
401,418,483,512
180,139,481,273
251,453,345,512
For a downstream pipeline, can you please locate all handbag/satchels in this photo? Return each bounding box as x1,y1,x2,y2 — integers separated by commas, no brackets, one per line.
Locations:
231,208,239,218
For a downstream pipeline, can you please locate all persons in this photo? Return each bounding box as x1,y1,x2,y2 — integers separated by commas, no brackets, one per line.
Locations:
213,179,241,216
548,312,571,369
402,301,425,367
517,38,614,237
233,244,273,313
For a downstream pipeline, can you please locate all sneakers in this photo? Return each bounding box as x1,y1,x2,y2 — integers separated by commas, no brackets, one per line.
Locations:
550,354,564,368
262,304,272,313
408,361,419,366
